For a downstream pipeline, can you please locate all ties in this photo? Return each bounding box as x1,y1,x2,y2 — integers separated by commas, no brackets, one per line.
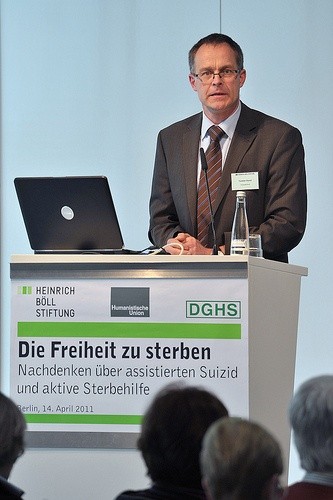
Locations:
197,125,225,246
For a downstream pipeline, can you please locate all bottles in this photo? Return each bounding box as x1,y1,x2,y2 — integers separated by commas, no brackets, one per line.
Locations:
230,191,249,257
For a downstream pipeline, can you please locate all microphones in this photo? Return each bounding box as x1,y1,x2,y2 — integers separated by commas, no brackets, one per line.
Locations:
200,148,218,255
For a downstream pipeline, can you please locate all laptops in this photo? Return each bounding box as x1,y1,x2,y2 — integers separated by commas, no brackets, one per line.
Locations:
14,176,142,254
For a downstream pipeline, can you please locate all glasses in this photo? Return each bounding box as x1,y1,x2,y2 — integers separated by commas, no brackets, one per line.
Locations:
192,69,241,81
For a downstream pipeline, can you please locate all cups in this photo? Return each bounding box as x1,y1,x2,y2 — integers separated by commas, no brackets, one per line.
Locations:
243,234,265,259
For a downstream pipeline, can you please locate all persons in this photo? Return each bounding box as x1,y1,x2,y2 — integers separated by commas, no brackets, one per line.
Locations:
146,33,308,264
0,376,333,500
